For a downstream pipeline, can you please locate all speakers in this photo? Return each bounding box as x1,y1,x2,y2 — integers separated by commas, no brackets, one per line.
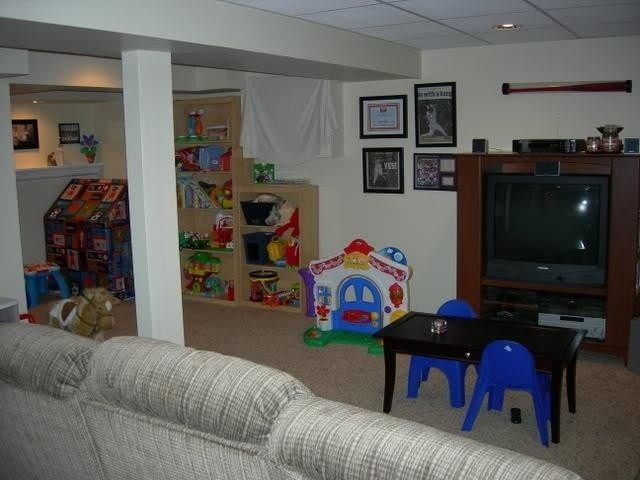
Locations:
472,139,488,154
624,137,640,155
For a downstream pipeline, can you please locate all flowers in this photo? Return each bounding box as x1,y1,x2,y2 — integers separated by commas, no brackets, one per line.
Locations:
77,132,100,154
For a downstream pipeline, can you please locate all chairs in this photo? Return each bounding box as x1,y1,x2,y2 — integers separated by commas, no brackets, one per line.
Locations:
406,297,481,409
460,338,552,447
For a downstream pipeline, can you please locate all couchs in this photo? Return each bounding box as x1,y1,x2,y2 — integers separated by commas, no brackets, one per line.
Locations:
0,321,585,479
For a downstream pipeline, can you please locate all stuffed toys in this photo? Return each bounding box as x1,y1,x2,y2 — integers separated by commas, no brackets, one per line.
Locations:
47,288,120,341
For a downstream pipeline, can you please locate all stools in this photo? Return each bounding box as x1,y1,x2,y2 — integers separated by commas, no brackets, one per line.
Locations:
22,260,71,310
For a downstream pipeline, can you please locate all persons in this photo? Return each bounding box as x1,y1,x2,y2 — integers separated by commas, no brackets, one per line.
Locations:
420,104,449,139
373,156,387,186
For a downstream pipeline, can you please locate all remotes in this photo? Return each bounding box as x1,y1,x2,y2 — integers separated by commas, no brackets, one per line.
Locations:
511,408,521,424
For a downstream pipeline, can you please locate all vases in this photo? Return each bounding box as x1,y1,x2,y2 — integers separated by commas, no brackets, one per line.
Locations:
86,154,95,165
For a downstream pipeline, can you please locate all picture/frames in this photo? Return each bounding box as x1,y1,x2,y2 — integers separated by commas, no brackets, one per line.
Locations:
10,117,40,150
358,94,409,140
58,123,81,145
412,80,459,148
412,151,456,192
361,147,406,194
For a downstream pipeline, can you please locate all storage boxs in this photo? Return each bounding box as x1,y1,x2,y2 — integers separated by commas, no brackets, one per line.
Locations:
0,296,20,322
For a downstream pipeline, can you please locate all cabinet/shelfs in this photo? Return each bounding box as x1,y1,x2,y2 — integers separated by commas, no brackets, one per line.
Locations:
171,95,259,309
236,181,321,316
452,151,640,368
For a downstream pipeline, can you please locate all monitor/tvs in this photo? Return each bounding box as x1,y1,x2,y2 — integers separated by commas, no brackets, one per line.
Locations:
483,174,609,286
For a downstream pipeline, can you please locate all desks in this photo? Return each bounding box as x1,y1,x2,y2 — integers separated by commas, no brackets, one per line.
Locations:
368,308,588,446
